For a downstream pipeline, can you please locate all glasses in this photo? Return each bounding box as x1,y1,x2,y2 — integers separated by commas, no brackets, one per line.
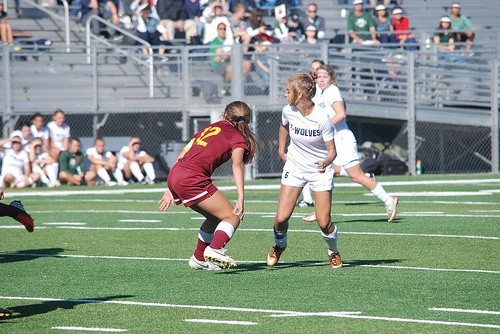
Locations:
308,10,316,13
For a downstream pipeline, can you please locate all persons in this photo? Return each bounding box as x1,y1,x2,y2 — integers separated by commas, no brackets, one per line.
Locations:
266,71,343,269
0,110,85,189
157,100,264,271
85,137,129,186
58,136,86,187
0,4,15,46
347,0,421,103
68,0,326,97
0,200,35,232
296,60,400,222
116,136,156,185
432,3,475,60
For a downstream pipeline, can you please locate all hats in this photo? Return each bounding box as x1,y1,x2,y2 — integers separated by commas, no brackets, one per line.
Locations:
392,9,403,14
375,4,386,10
353,0,363,6
441,17,451,22
451,1,461,9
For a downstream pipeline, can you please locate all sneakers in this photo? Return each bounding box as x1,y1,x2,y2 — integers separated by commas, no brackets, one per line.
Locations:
203,245,237,269
302,214,316,222
188,255,221,271
267,244,287,266
328,252,342,269
385,196,399,222
295,199,315,207
10,200,35,233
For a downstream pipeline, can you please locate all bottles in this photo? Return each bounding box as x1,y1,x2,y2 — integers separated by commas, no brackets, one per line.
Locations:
416,159,422,176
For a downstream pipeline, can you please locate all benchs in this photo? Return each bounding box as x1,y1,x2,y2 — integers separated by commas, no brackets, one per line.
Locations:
0,0,500,104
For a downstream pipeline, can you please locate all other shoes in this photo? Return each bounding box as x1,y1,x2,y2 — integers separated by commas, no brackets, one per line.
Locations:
47,180,61,188
119,181,128,186
153,178,160,184
106,181,115,187
140,178,148,185
